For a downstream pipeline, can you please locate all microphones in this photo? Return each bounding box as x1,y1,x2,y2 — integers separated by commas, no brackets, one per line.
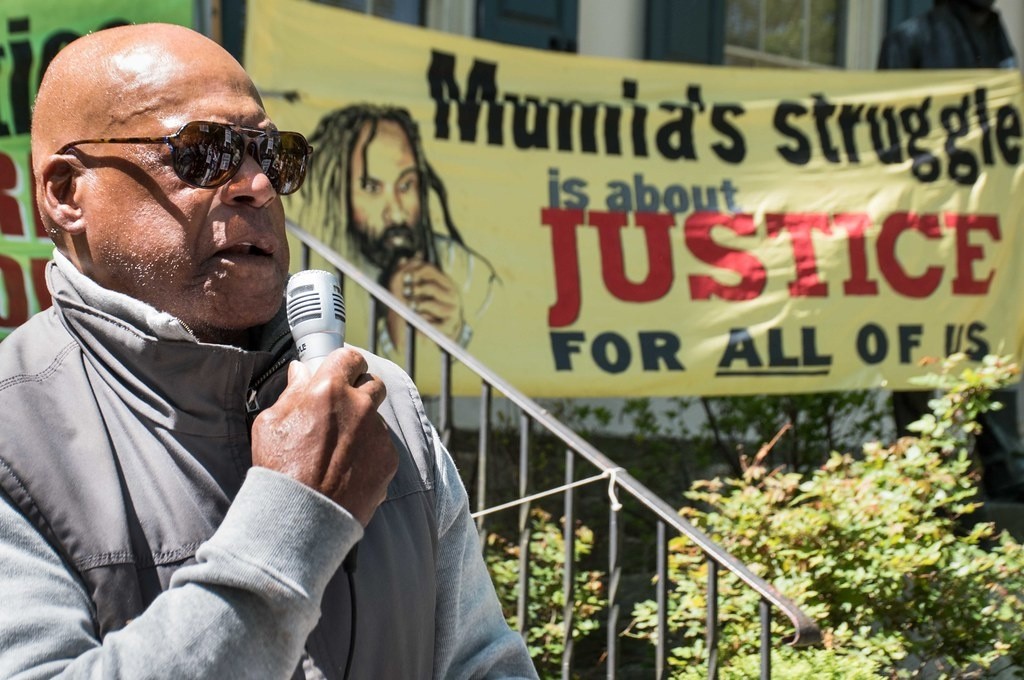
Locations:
285,268,347,377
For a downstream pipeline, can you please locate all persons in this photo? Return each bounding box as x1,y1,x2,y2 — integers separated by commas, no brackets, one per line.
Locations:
174,134,235,182
293,104,506,362
263,148,301,194
0,22,540,680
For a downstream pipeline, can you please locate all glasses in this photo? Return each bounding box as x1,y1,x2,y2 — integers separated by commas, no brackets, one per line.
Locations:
57,121,313,196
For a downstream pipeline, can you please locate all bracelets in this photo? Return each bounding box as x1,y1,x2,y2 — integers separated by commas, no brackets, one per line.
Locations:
448,322,473,362
378,327,396,358
